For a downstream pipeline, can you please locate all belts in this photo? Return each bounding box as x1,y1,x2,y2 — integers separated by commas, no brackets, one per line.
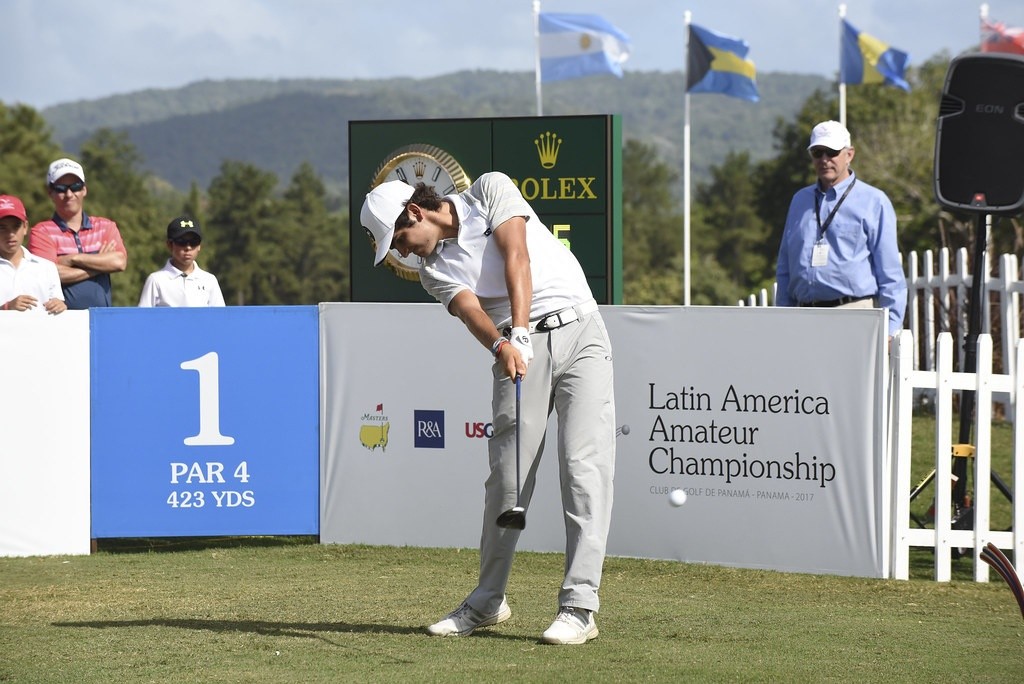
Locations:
495,298,600,340
800,296,866,308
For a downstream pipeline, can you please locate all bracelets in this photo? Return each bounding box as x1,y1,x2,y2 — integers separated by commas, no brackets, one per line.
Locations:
491,337,512,359
5,301,9,309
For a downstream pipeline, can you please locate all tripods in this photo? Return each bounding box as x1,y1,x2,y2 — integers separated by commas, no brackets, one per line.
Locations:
904,210,1024,558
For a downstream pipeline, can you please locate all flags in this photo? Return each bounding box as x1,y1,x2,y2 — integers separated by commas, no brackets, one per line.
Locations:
981,20,1024,56
684,24,759,104
535,11,631,80
838,18,910,91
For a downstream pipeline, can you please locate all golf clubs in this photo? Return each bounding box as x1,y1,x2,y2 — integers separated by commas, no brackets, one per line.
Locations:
495,375,526,532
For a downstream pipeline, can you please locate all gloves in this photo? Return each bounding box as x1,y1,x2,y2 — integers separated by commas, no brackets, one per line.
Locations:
509,326,534,369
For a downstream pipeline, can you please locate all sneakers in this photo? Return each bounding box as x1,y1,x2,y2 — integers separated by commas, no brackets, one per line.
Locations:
425,593,512,637
542,607,598,644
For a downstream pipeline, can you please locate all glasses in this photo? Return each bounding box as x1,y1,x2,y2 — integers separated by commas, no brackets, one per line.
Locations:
53,180,84,193
811,147,842,158
174,236,201,247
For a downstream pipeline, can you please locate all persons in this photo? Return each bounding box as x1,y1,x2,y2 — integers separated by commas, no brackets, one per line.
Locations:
0,194,69,315
774,120,908,347
361,172,616,647
28,159,127,310
138,217,226,308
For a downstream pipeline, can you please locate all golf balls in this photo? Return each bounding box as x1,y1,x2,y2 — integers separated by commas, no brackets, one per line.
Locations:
669,488,687,508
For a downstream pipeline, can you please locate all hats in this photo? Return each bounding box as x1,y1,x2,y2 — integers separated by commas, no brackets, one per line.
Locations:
0,193,27,223
359,179,416,267
168,216,203,240
806,120,851,151
46,157,86,187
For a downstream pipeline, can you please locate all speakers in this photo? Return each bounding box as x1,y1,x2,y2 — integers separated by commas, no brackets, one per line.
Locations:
933,54,1024,221
